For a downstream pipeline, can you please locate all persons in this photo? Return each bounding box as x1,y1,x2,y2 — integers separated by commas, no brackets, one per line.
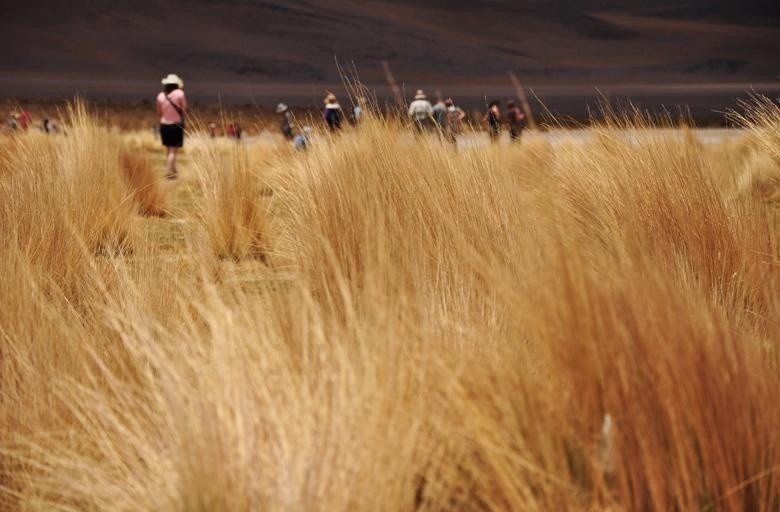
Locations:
323,94,345,133
155,73,189,179
294,126,315,153
208,120,241,139
408,89,433,135
349,98,366,128
433,97,528,146
275,102,295,141
5,109,59,137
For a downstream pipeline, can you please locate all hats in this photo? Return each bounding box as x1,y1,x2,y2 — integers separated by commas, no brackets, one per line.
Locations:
414,89,426,99
276,103,288,113
161,74,184,89
324,94,337,104
